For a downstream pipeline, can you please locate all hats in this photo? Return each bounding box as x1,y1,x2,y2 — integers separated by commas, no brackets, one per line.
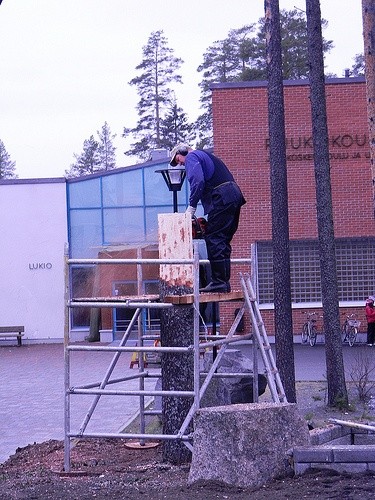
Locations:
369,296,375,302
169,143,188,167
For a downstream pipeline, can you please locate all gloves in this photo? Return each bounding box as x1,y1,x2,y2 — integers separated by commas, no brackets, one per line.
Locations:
185,205,196,221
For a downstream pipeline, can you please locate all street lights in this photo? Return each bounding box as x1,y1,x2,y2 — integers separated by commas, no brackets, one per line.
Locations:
154,159,188,214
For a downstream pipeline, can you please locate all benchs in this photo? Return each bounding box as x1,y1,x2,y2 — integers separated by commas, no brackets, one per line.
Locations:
0,326,26,345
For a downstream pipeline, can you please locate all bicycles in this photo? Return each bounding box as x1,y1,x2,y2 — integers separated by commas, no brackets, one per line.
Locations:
301,310,319,347
340,311,361,347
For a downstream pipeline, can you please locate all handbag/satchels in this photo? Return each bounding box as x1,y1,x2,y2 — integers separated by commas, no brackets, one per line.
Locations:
192,216,207,239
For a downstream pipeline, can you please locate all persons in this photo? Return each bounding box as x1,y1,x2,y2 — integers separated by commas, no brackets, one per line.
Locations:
365,296,375,346
169,145,247,293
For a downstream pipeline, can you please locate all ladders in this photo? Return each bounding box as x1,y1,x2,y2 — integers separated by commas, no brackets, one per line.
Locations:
238,272,288,404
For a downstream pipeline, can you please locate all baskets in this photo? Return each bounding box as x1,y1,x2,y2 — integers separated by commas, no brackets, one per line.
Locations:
347,318,361,327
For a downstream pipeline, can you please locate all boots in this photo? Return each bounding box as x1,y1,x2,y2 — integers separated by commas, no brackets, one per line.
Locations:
226,259,231,293
199,259,227,293
